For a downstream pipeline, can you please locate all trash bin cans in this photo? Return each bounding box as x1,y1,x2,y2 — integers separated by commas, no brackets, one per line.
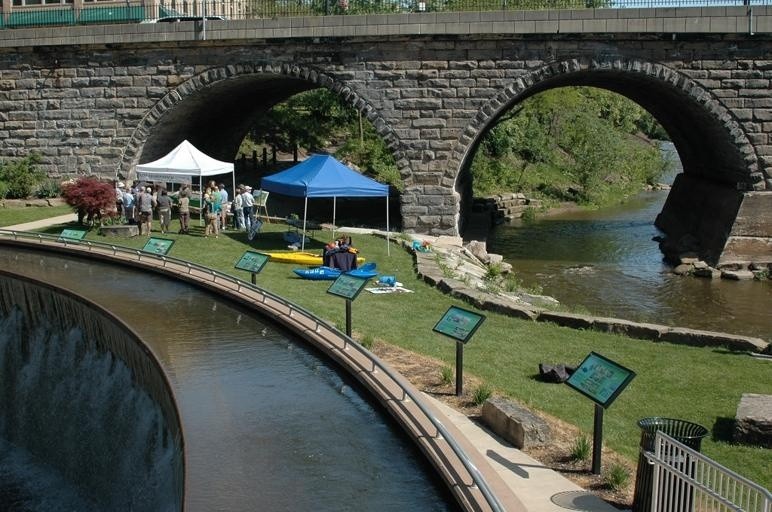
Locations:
632,417,707,512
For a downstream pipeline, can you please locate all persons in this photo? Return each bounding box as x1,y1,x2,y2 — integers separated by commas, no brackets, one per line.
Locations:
85,179,258,241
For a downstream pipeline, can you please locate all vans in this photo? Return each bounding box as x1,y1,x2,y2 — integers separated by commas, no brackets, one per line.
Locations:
143,14,226,25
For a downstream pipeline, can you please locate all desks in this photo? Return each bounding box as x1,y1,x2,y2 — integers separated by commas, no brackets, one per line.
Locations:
285,219,322,240
